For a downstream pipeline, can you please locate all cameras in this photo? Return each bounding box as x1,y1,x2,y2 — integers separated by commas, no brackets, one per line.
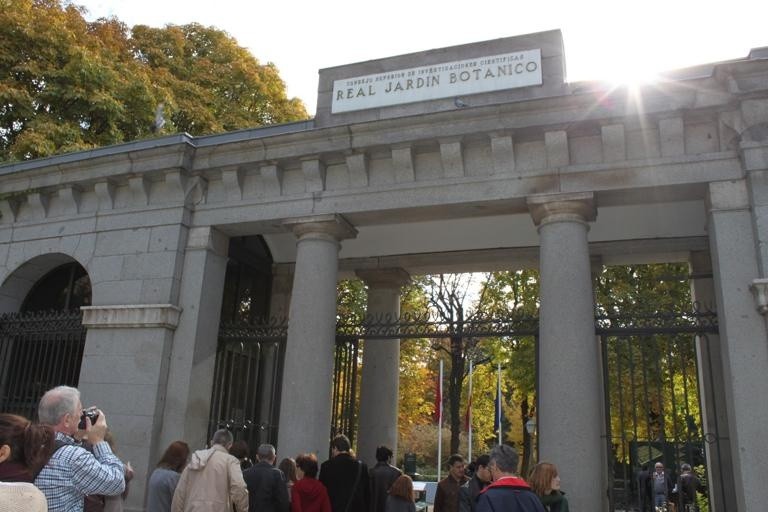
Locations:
77,409,99,429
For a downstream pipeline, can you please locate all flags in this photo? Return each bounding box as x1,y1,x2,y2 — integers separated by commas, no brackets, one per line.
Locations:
463,395,472,434
493,380,499,434
432,372,442,424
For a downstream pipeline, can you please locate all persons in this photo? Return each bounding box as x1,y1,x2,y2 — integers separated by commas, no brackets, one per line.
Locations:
144,429,417,512
0,385,135,511
433,446,568,512
636,460,708,512
